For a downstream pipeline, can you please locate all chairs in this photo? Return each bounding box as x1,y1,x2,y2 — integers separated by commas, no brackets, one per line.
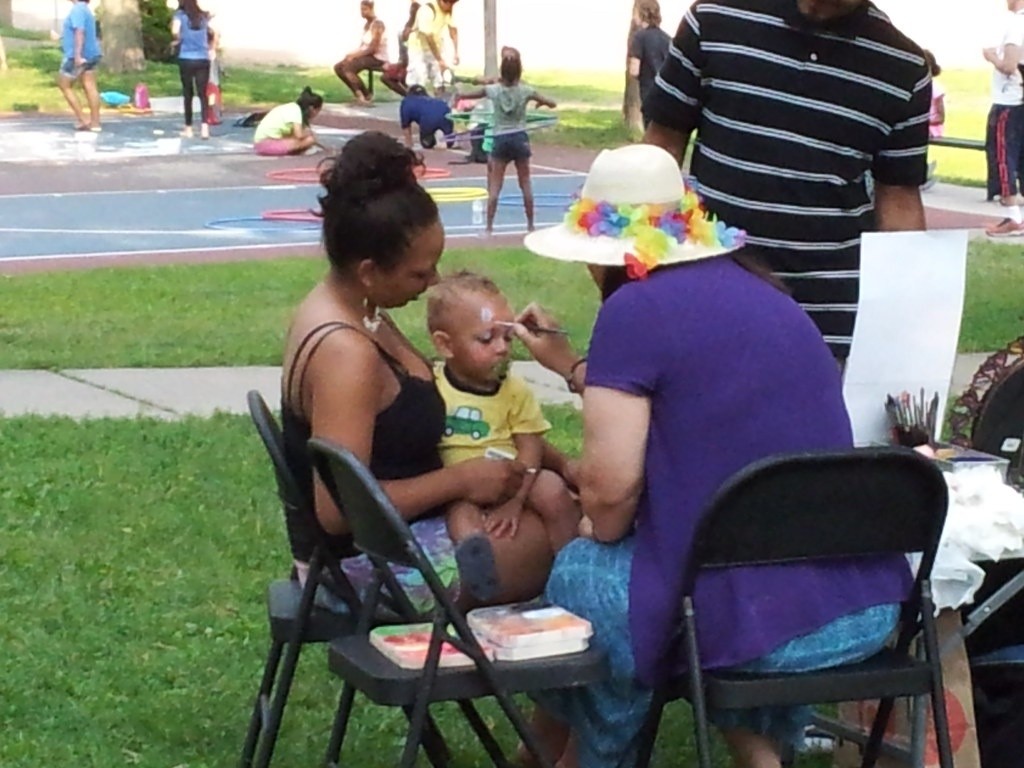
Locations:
636,445,953,768
245,389,608,768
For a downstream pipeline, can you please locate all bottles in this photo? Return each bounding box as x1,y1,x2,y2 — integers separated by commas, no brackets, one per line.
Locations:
100,91,131,105
472,197,482,225
134,84,149,108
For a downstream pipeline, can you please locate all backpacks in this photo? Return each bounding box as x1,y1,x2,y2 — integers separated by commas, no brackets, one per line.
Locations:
402,1,436,41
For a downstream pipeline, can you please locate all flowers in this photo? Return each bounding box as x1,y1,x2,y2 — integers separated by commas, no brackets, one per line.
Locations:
564,176,747,278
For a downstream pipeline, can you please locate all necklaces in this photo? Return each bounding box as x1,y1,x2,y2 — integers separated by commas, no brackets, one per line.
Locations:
361,306,383,333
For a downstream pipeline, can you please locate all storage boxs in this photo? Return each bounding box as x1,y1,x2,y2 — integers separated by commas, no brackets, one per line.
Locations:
913,442,1010,484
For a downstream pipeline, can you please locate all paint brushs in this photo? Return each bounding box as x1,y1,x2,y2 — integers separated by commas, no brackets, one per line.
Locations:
494,321,568,334
884,388,938,447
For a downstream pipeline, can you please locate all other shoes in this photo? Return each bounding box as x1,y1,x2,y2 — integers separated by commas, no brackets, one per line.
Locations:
1000,193,1024,206
457,535,500,600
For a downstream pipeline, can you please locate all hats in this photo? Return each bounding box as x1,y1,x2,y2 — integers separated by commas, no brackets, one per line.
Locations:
522,143,747,281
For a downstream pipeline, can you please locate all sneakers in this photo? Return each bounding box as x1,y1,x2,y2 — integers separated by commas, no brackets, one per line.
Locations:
986,218,1024,237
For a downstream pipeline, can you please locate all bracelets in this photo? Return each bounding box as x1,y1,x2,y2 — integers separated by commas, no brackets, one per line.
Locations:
565,358,589,392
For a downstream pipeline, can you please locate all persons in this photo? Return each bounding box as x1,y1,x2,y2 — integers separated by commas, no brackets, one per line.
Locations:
253,85,324,155
517,142,916,768
381,0,460,96
56,0,104,133
456,46,556,239
280,129,580,621
400,83,454,147
171,0,221,139
630,0,670,103
982,0,1024,238
332,1,388,103
640,0,945,373
